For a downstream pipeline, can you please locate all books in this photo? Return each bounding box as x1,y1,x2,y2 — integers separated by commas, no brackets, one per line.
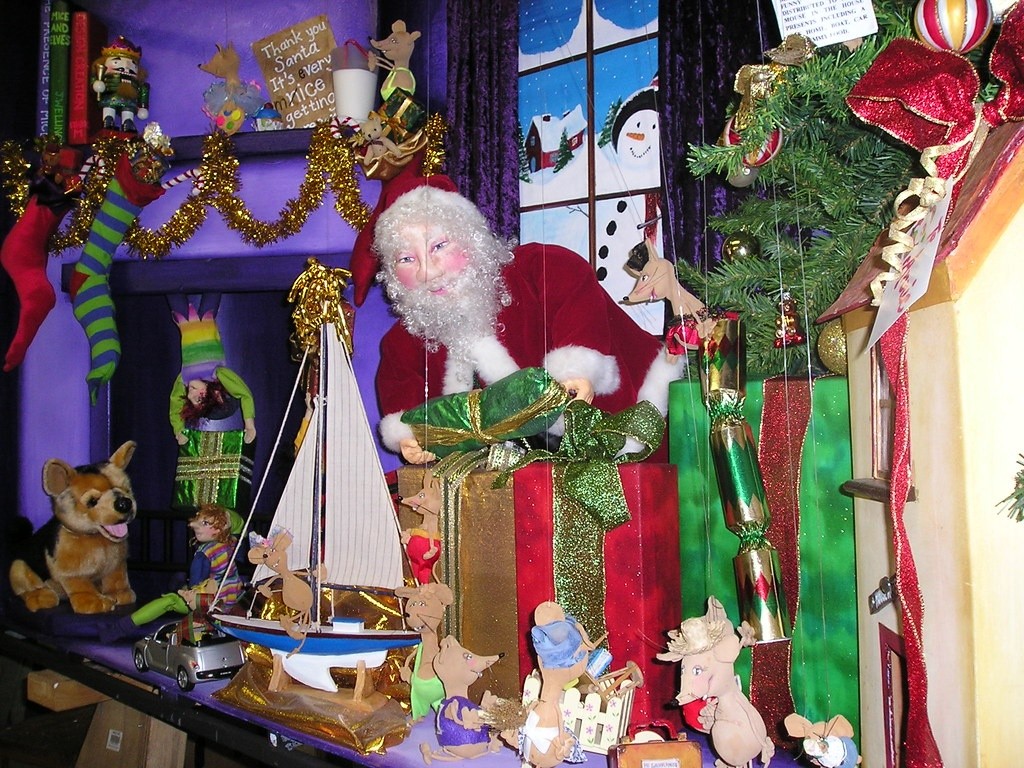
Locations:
48,0,71,143
36,0,51,138
69,11,108,145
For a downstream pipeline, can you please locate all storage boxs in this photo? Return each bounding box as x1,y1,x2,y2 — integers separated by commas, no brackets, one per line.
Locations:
668,376,861,753
608,740,703,768
171,428,257,515
396,462,684,741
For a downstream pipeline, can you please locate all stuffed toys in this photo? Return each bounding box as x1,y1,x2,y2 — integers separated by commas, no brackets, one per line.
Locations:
8,440,137,614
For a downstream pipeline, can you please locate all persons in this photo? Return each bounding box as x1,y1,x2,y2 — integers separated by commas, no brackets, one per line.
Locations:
99,506,245,645
169,303,258,445
353,174,689,464
89,36,150,131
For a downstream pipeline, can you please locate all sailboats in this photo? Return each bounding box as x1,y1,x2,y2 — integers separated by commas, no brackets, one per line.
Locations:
202,316,426,656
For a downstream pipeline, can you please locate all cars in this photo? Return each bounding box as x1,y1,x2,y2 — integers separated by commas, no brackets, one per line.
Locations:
131,620,247,692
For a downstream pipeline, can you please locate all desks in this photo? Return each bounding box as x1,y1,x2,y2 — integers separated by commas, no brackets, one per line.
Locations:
0,619,812,768
